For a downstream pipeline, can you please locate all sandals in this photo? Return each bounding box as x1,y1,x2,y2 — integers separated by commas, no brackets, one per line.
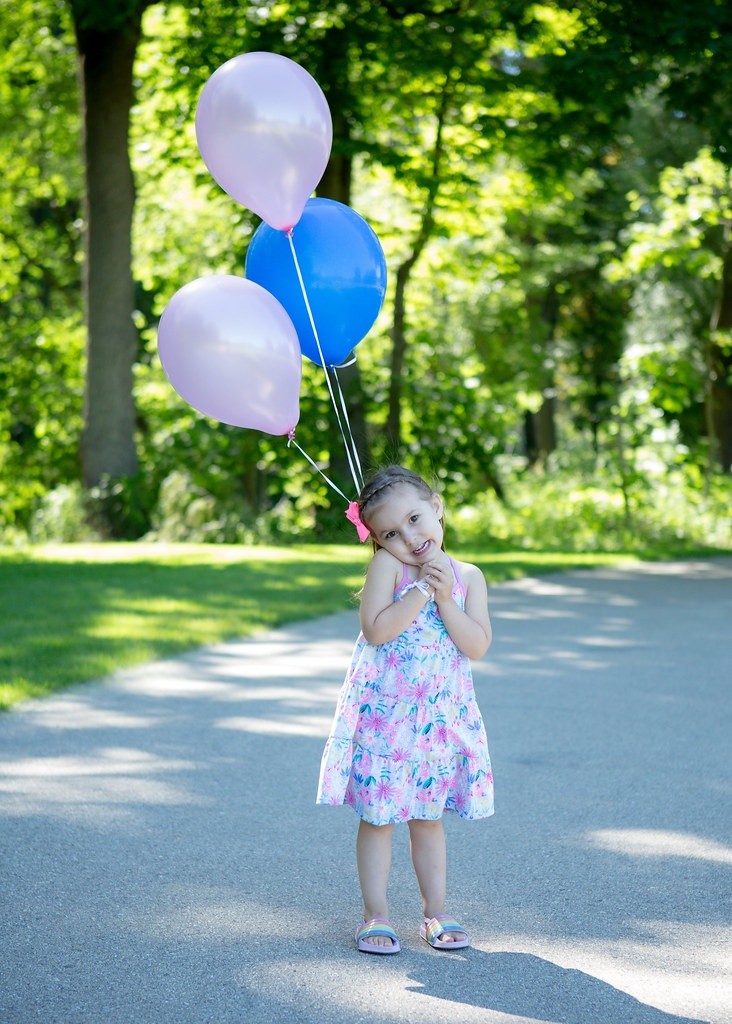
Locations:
354,917,400,953
419,915,469,948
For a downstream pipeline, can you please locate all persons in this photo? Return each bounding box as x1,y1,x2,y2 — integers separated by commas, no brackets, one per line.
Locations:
315,465,494,954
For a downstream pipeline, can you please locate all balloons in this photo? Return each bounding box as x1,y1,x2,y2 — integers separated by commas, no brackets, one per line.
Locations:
195,52,333,236
157,275,302,439
244,197,388,372
